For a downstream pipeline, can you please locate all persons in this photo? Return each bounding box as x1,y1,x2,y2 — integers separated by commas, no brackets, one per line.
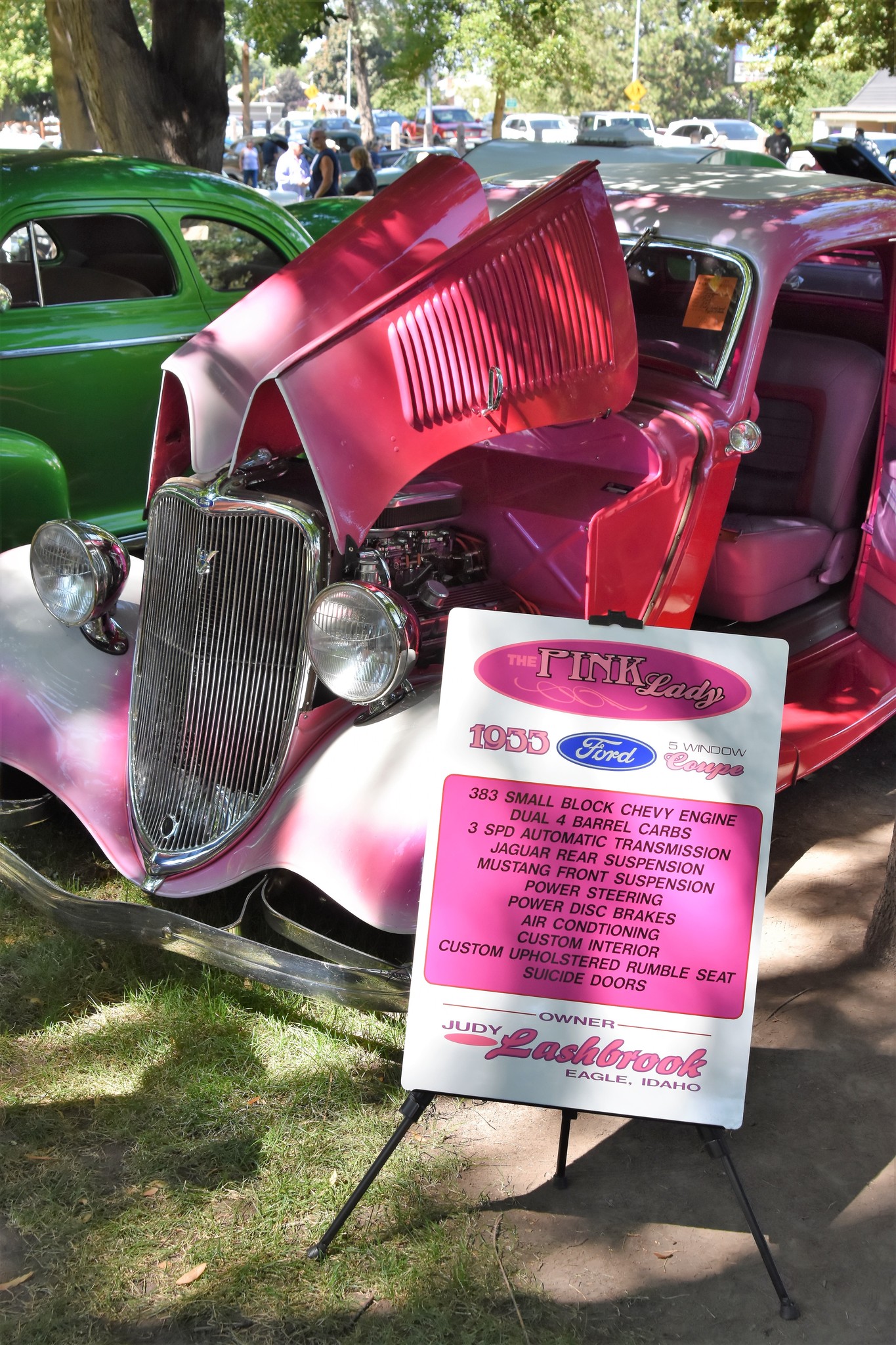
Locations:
239,139,260,189
764,120,793,166
689,129,702,144
273,130,388,205
854,127,880,161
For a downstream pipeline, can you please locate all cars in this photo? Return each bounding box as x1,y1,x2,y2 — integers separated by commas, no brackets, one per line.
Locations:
501,112,578,144
658,118,772,154
222,111,461,206
479,112,513,130
803,136,896,187
0,149,896,1013
0,149,378,556
354,109,412,143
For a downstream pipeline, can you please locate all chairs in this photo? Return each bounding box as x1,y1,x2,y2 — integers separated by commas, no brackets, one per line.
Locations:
702,334,884,625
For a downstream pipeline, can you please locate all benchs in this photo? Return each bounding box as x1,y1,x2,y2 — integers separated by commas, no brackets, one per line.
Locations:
35,265,156,304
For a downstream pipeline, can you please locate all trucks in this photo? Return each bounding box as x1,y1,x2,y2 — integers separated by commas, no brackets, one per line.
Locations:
566,110,659,145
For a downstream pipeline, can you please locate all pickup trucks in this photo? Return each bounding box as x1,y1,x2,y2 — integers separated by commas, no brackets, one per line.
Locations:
404,105,488,142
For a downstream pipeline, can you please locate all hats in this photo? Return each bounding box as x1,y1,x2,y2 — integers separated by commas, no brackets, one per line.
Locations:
287,132,307,145
774,120,783,129
